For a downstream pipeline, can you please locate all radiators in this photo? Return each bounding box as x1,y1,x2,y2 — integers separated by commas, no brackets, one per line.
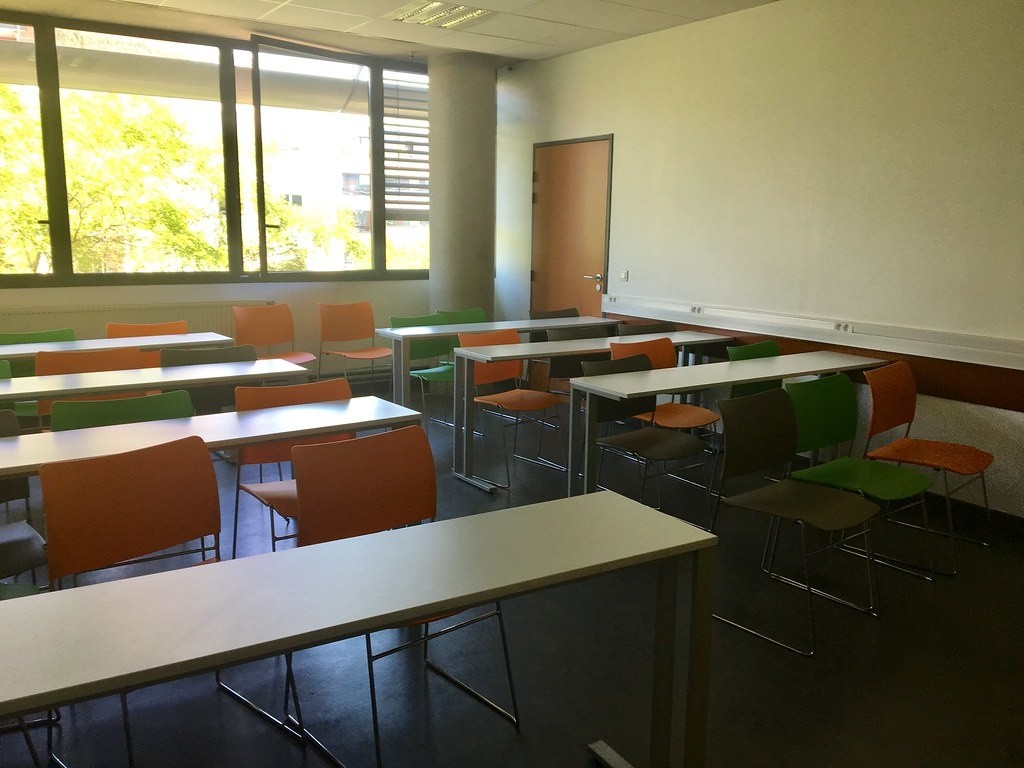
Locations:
0,301,233,339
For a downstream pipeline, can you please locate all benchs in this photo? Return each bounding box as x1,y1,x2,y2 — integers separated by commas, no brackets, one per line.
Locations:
0,489,718,768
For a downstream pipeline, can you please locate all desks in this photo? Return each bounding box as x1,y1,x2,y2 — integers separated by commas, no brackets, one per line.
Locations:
0,395,423,480
450,329,734,493
0,358,307,402
375,316,622,407
566,351,895,495
1,330,235,359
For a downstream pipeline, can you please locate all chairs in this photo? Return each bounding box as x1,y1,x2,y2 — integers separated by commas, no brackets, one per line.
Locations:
713,388,881,656
716,340,795,483
761,374,936,604
618,321,676,404
581,354,712,533
611,338,707,486
537,327,608,472
841,361,994,576
0,301,577,762
